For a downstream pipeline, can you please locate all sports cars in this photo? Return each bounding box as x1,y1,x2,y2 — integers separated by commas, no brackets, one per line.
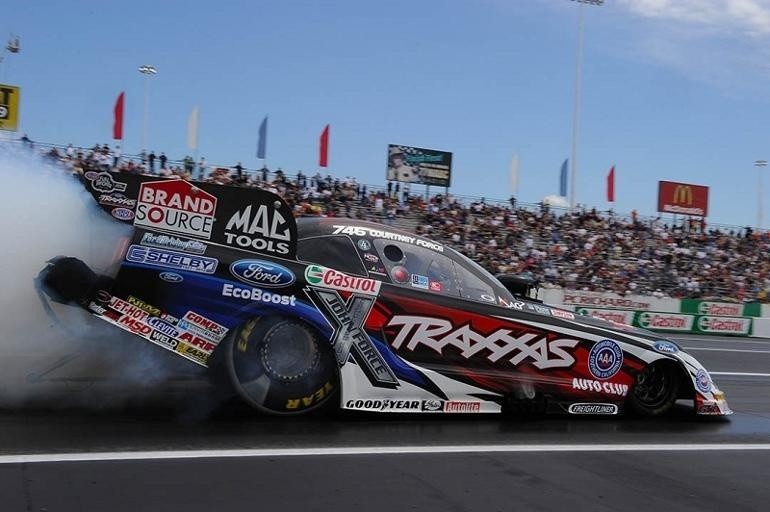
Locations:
30,178,734,427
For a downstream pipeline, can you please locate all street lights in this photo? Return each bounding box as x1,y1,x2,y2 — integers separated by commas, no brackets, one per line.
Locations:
138,63,158,152
754,159,767,234
567,0,605,213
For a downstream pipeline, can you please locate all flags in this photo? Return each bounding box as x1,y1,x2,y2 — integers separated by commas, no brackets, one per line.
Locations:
557,159,567,197
320,124,329,169
508,151,518,194
187,104,198,153
112,90,124,140
606,164,615,204
256,115,266,161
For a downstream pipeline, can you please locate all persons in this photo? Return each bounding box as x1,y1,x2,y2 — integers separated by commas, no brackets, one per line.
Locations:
389,180,769,304
21,134,33,150
39,143,384,224
385,180,768,305
388,145,423,183
